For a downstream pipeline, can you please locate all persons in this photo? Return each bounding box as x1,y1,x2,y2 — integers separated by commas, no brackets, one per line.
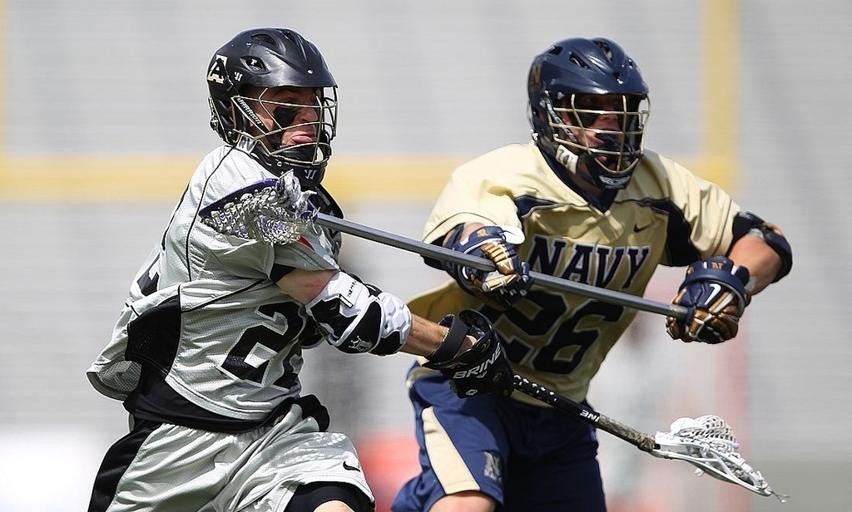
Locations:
390,36,793,512
84,26,516,512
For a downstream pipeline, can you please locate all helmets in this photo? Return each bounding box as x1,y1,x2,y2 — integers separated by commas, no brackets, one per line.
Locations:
207,28,338,184
527,38,651,200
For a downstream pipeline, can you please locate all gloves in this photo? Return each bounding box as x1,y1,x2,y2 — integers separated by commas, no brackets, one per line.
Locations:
421,308,513,398
666,256,751,344
441,224,535,311
